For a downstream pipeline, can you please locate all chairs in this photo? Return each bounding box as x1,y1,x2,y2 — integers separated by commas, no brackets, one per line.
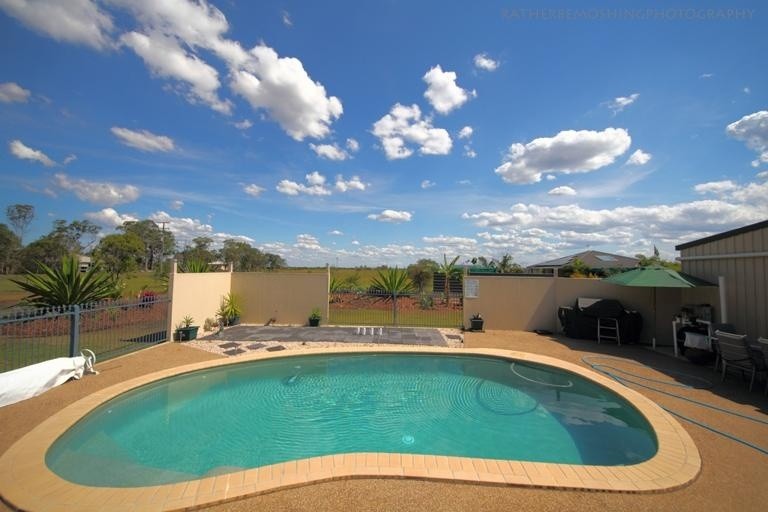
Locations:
714,331,767,393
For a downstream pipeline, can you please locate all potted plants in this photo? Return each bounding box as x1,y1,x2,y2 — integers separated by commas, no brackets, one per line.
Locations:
308,306,323,327
176,316,199,341
469,312,485,332
215,289,243,327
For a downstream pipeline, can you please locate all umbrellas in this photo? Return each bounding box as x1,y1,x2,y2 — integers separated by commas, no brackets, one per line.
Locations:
598,257,718,350
